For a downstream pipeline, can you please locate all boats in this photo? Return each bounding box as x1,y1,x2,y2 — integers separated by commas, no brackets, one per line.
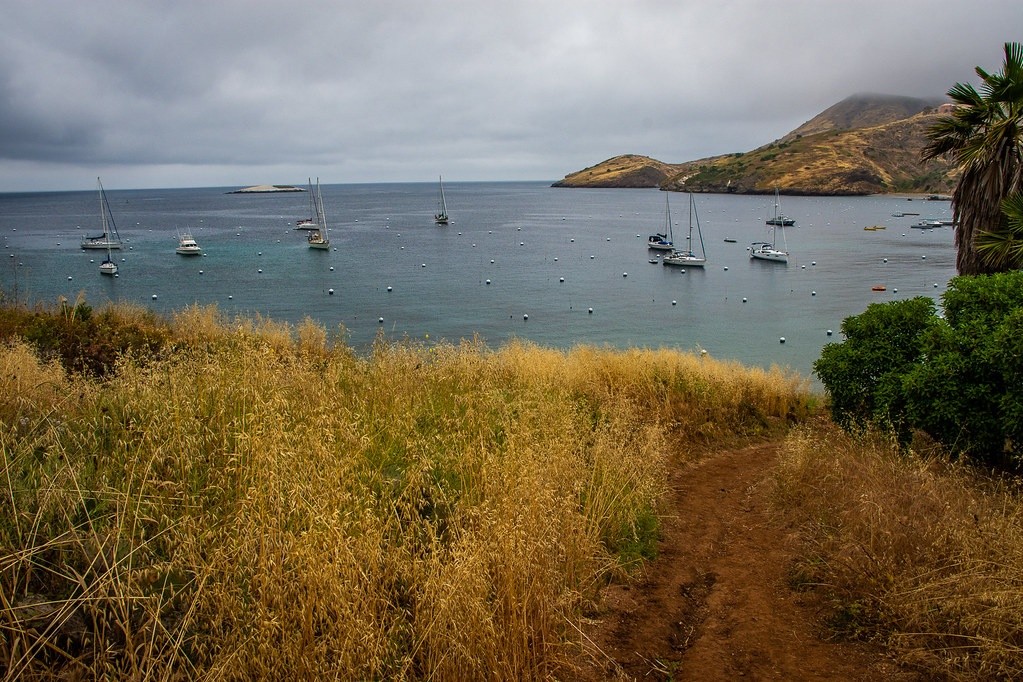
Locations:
175,223,202,256
910,217,961,228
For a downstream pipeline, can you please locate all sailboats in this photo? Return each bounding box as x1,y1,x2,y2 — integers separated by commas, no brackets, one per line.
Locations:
746,185,790,263
661,190,707,267
98,202,119,275
80,177,124,249
306,178,332,249
647,186,676,250
291,178,320,230
434,175,451,223
765,186,796,226
